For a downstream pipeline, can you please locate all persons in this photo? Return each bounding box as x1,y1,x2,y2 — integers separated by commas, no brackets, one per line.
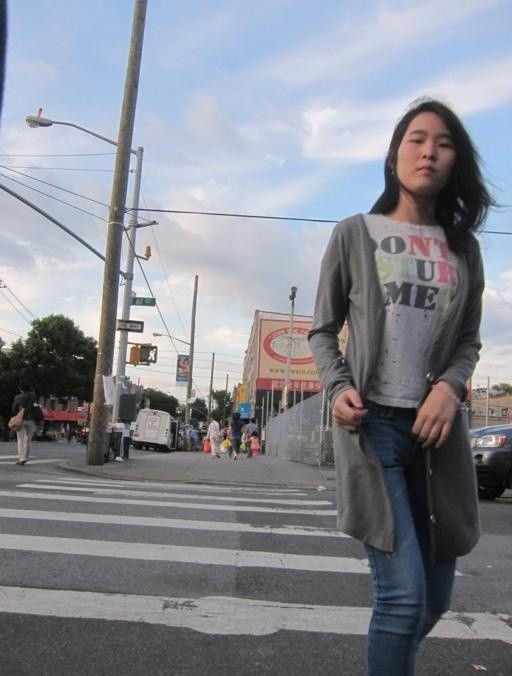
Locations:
302,90,510,675
9,383,42,466
190,412,261,460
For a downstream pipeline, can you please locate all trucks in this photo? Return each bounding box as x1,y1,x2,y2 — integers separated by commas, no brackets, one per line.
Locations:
131,407,179,453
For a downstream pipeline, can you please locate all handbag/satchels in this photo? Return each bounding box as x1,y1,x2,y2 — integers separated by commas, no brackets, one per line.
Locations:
8,407,27,431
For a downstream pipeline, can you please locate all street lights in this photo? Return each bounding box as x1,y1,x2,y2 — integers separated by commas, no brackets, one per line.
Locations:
283,286,298,410
26,113,143,464
152,332,194,452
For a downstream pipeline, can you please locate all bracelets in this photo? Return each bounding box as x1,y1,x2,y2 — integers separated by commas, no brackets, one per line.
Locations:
429,380,462,407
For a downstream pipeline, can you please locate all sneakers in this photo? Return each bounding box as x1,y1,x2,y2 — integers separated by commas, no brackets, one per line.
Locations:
16,460,27,465
233,454,237,460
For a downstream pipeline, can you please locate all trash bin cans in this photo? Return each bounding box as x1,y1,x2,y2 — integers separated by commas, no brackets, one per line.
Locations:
108,432,131,461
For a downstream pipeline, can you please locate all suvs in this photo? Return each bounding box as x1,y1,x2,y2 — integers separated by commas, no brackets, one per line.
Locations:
469,421,511,501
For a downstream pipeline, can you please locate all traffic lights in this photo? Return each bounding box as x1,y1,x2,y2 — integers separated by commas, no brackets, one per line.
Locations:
140,345,157,364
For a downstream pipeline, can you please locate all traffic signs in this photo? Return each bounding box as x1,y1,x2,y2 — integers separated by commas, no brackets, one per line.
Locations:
117,319,144,332
129,297,156,306
